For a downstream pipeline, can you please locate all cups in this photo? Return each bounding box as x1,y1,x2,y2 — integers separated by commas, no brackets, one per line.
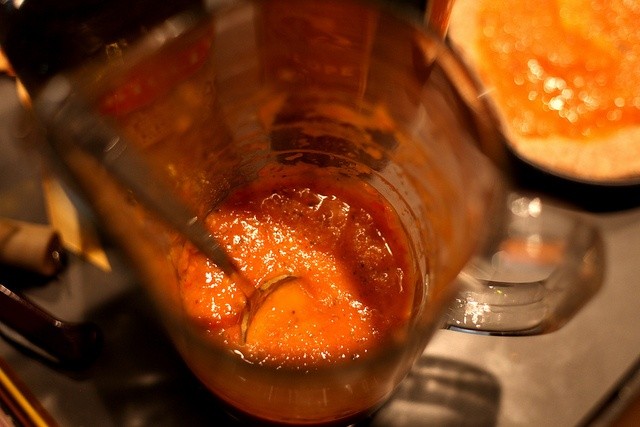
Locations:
85,6,607,425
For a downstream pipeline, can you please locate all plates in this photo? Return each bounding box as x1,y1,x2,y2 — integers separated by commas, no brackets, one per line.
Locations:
448,1,639,184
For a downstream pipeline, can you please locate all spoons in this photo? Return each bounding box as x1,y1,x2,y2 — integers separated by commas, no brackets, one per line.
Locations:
54,95,303,341
